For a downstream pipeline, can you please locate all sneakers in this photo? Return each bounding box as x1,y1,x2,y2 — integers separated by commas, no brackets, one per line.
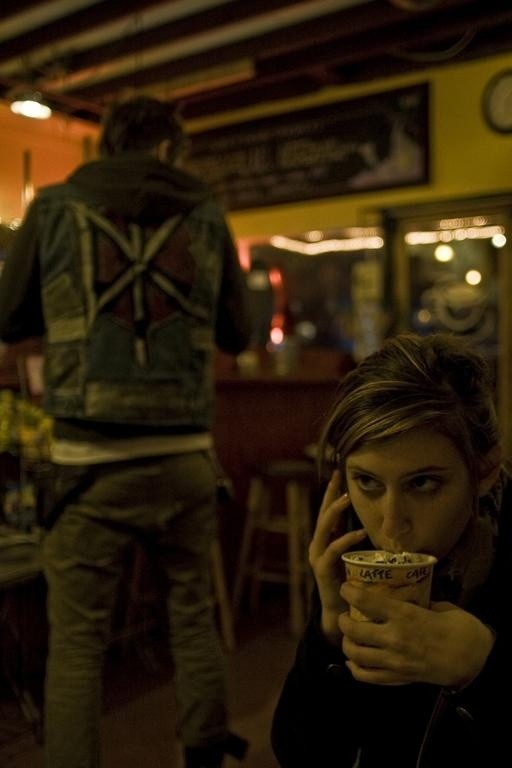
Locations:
185,731,248,767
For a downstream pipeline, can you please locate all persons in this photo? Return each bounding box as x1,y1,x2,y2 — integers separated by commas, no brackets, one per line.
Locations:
269,334,512,768
0,90,269,768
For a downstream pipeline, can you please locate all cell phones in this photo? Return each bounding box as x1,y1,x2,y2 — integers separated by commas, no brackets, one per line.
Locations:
338,459,376,551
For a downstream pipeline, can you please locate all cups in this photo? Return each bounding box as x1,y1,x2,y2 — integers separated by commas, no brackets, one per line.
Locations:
342,549,439,621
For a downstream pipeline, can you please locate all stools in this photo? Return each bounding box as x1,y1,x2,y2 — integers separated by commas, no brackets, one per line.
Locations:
120,461,315,652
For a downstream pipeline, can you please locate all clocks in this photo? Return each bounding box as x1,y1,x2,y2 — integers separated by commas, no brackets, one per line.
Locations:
482,66,511,134
